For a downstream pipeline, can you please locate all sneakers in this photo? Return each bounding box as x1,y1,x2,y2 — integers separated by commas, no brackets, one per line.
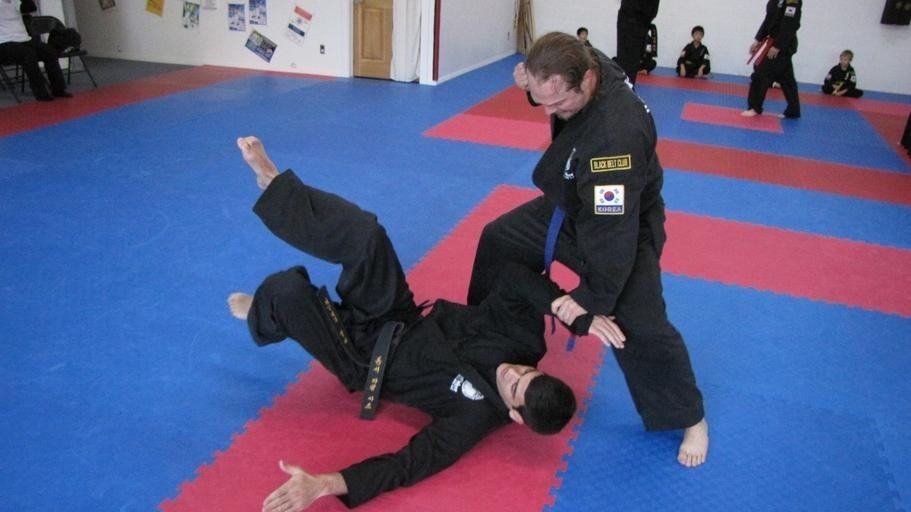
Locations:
34,87,55,101
51,87,75,99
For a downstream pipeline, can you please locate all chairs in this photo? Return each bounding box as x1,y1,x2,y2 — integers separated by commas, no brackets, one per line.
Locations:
1,10,100,103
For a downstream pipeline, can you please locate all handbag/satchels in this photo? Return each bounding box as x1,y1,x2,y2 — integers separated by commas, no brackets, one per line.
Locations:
47,27,81,55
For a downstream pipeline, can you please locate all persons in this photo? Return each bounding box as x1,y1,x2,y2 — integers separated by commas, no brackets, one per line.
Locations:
0,0,74,102
576,26,592,48
819,48,864,98
675,24,711,79
738,0,803,120
233,134,627,512
615,0,661,86
462,29,713,470
